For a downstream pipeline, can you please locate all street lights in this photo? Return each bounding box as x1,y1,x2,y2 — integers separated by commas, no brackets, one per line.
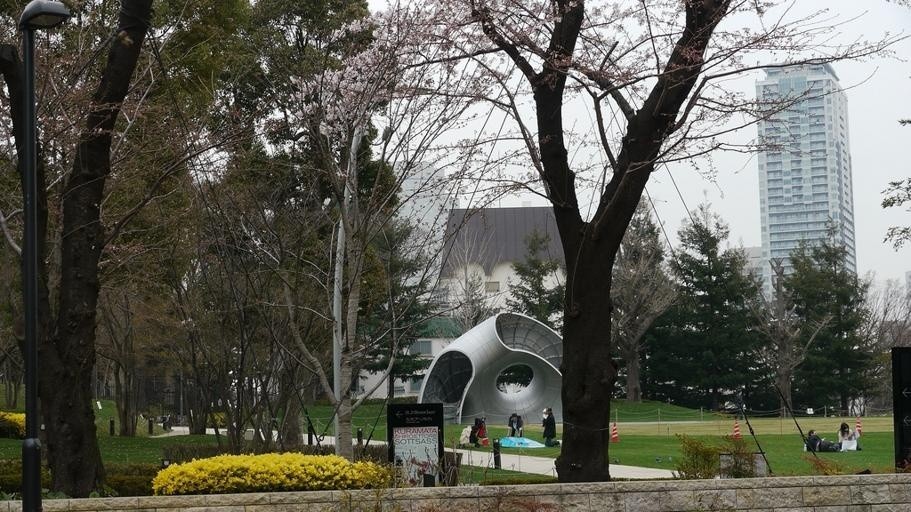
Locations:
17,0,71,511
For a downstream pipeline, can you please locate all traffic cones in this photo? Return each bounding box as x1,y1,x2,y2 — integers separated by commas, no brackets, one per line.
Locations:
612,423,620,442
731,420,743,439
856,419,861,435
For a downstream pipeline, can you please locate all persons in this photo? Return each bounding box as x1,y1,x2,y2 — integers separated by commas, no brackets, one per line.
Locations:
161,413,167,431
472,418,488,438
838,422,863,450
541,408,563,447
806,429,840,452
165,414,173,430
507,412,524,437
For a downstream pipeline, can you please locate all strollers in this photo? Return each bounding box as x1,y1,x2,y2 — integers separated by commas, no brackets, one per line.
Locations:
457,416,487,449
